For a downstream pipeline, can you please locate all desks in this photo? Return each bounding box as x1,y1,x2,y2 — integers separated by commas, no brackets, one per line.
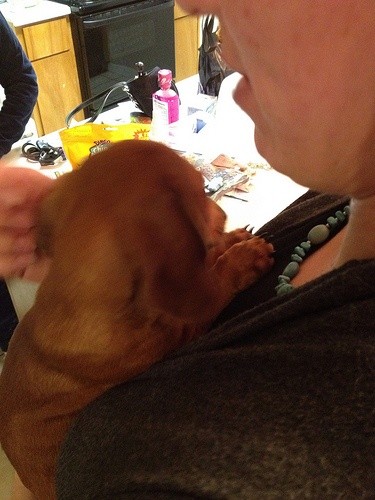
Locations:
0,75,351,326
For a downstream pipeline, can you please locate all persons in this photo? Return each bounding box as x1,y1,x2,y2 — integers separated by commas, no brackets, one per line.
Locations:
0,0,375,500
0,10,39,358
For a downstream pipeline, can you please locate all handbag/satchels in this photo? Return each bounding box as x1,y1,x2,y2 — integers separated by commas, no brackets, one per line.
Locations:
198,13,233,96
66,61,179,128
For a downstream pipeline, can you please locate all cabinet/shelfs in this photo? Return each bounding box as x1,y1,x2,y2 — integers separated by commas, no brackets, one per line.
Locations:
0,0,85,139
174,0,219,83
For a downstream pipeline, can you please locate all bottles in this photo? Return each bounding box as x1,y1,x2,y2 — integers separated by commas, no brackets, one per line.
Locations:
153,69,179,143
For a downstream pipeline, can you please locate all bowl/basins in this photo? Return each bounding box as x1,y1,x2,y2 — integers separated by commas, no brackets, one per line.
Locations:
59,123,150,171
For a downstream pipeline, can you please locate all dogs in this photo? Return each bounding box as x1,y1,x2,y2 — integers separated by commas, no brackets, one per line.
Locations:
1,139,275,499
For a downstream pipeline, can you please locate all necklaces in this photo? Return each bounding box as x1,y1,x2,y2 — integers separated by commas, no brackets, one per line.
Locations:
276,207,351,295
